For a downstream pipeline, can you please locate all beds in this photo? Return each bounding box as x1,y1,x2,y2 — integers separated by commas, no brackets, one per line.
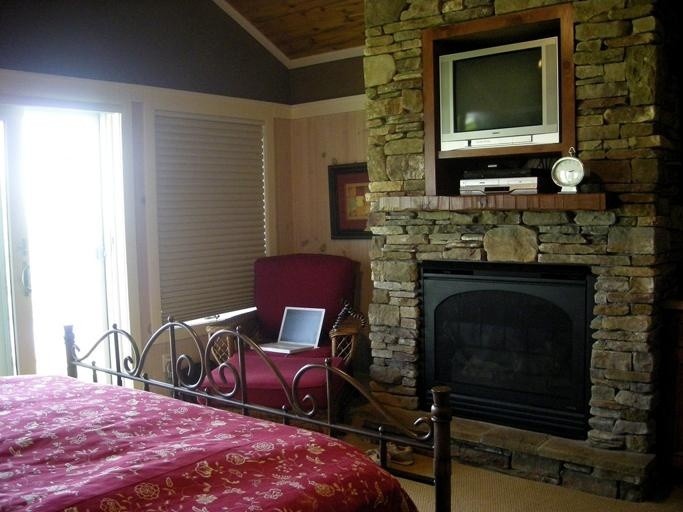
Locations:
0,314,453,512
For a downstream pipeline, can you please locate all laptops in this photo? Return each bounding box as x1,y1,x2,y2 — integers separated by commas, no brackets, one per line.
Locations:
250,306,328,358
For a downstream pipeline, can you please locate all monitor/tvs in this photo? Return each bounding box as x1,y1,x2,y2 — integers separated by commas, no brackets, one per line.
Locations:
437,35,560,152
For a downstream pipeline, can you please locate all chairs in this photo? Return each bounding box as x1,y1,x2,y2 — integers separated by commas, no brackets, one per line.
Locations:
197,252,362,429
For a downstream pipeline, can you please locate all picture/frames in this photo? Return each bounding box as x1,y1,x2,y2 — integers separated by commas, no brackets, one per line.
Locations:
327,162,373,239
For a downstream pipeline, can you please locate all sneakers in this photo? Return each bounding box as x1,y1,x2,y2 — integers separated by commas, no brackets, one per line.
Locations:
365,448,392,469
374,442,415,467
